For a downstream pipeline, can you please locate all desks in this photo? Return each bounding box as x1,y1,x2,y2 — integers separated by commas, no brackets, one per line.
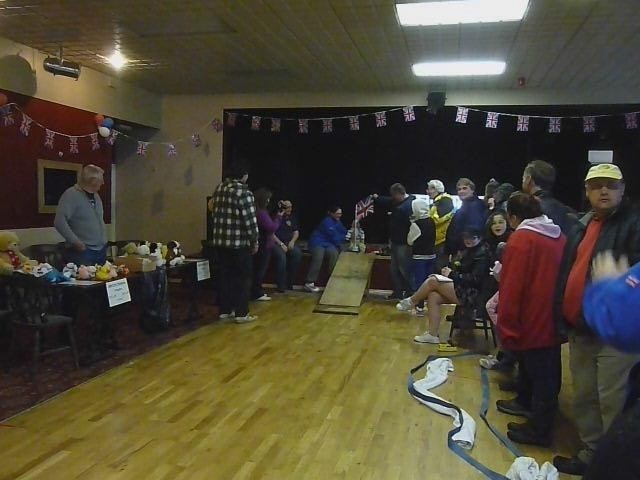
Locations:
53,253,206,355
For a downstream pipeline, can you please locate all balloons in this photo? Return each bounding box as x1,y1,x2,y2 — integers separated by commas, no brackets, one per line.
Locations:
102,114,115,126
98,125,111,139
95,111,104,126
0,93,8,105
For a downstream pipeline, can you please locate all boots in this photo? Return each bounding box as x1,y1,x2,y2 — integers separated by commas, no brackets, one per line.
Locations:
506,416,554,448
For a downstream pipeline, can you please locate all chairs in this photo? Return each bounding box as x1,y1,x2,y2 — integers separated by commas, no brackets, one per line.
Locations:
99,238,156,259
448,298,499,349
1,277,82,376
27,244,62,270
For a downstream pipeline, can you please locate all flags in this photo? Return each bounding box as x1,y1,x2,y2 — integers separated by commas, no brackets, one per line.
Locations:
295,117,309,134
372,110,388,127
322,117,333,132
0,105,15,128
105,130,120,147
347,114,358,130
486,111,502,130
165,142,176,157
357,194,375,218
209,115,225,134
401,104,414,123
42,129,57,151
623,110,639,128
515,114,530,132
582,116,597,134
18,113,32,137
227,111,237,128
271,117,281,135
68,136,80,154
548,117,559,135
135,139,148,158
191,130,201,147
249,115,261,130
90,133,102,152
455,106,469,124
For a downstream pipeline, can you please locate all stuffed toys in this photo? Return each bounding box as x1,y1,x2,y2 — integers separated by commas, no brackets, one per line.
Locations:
32,260,131,283
0,228,31,276
120,238,186,268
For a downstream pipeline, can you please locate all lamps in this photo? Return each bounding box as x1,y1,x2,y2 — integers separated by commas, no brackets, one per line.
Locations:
394,0,533,25
109,52,132,75
40,40,85,81
409,60,514,79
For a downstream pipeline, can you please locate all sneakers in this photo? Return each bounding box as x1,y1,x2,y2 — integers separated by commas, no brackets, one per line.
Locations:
258,293,272,303
219,312,259,323
414,332,441,344
497,397,531,415
384,292,405,302
303,282,321,293
395,297,416,312
498,379,521,392
479,354,500,370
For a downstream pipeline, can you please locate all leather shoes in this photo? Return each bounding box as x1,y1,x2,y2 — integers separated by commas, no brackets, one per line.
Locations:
553,455,589,475
278,282,295,293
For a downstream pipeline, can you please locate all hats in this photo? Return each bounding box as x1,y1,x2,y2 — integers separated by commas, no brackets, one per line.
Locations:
459,225,482,236
584,163,623,182
223,157,258,178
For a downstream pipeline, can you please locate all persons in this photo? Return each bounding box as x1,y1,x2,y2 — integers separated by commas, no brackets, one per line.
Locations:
305,202,351,293
255,188,303,300
209,158,261,324
52,163,108,271
